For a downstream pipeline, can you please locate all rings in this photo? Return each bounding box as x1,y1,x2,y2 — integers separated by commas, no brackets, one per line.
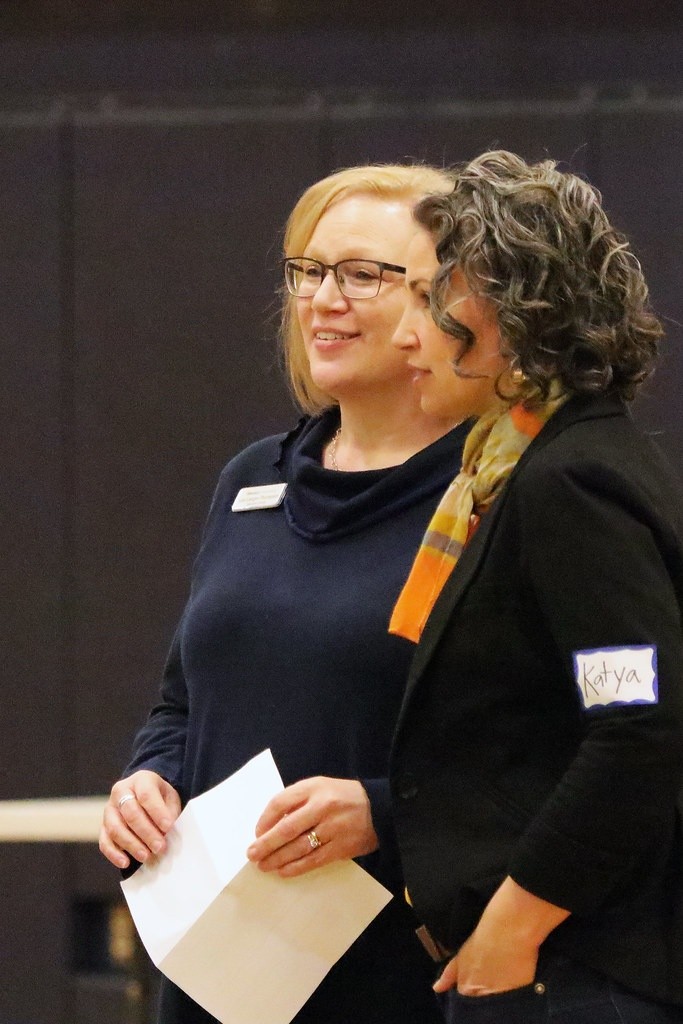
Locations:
306,830,322,848
118,793,135,807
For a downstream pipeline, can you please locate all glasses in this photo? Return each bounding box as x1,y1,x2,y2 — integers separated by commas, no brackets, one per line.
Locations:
282,256,410,299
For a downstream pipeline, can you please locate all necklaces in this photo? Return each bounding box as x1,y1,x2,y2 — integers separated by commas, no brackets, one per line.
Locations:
329,428,341,471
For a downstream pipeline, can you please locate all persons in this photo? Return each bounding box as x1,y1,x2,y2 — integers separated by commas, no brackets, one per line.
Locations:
393,149,683,1024
96,163,481,1024
